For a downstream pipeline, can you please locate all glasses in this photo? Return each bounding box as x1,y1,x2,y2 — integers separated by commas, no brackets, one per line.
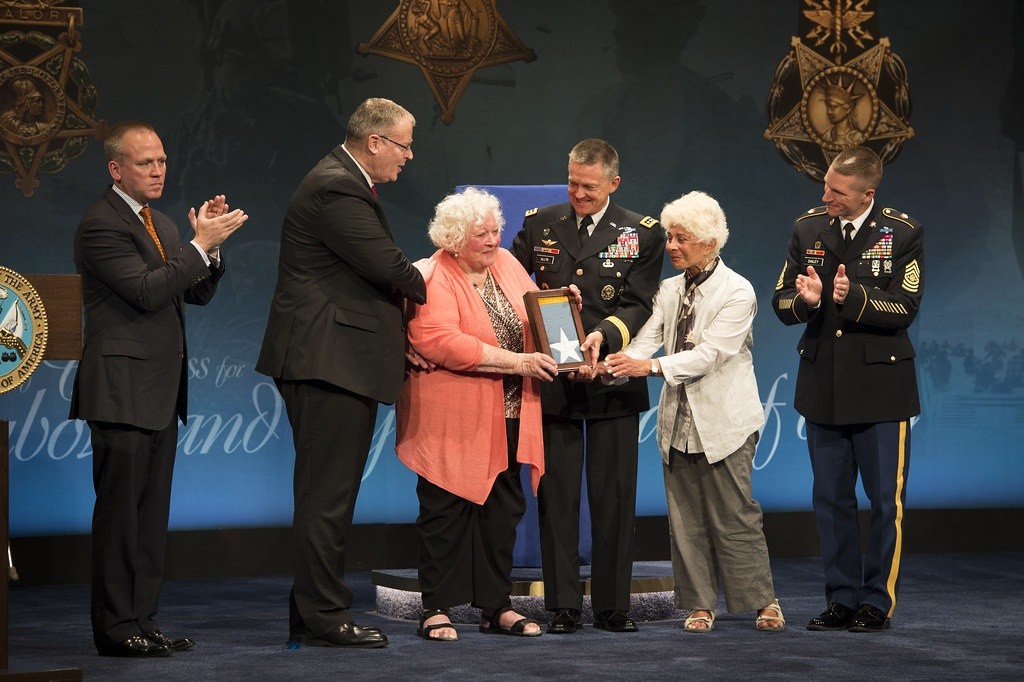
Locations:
376,135,412,151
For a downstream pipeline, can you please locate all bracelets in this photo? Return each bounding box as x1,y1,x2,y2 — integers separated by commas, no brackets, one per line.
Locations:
651,359,658,376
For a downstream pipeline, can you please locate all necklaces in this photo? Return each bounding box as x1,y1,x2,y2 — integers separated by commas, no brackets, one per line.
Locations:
473,270,503,315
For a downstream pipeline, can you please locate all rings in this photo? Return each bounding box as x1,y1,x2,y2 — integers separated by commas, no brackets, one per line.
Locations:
217,213,221,216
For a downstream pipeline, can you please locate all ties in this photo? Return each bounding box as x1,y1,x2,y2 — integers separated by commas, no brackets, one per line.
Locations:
844,223,855,247
578,215,593,248
140,206,167,264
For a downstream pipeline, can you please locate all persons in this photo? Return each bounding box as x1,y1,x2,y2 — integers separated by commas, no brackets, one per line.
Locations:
397,188,582,639
774,147,926,631
567,190,784,631
68,122,248,658
255,98,437,651
508,138,669,634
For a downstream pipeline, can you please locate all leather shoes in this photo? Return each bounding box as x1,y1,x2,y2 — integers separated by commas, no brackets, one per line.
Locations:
549,609,581,633
289,621,389,648
807,602,857,630
850,610,890,632
144,631,194,650
593,610,637,631
99,634,171,657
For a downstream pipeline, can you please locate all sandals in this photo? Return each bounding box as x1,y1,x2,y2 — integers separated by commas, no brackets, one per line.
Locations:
480,607,542,636
417,609,458,641
685,610,714,633
756,598,785,631
